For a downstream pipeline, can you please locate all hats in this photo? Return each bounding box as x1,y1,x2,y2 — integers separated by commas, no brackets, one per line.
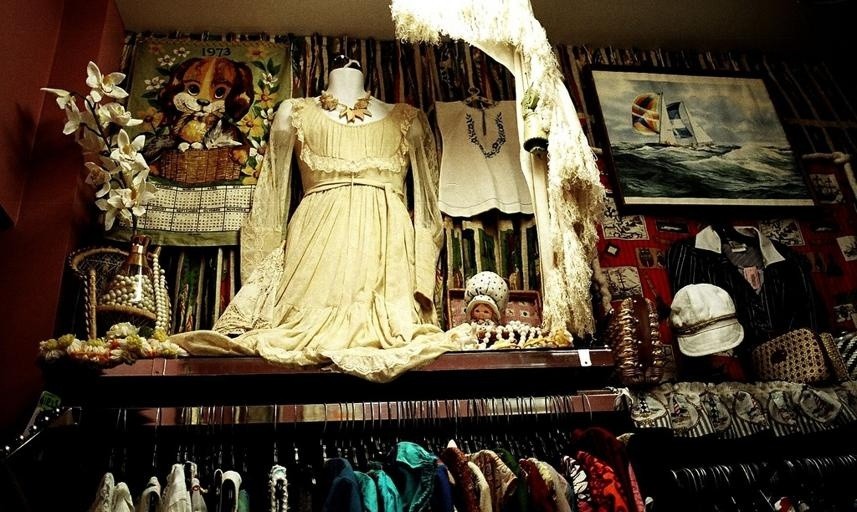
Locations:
672,284,744,358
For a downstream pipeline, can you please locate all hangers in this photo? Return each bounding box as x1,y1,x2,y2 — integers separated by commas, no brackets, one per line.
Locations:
712,222,759,248
461,87,498,105
103,405,248,507
670,454,857,512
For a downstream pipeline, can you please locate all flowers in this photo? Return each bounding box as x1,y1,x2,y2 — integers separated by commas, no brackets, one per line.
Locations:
236,44,282,185
40,61,150,276
131,40,191,141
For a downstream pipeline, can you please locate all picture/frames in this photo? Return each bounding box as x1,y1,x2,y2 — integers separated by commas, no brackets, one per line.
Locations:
580,63,821,219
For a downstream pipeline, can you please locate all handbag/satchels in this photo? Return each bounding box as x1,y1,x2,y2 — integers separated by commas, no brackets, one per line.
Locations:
752,328,851,385
610,293,665,385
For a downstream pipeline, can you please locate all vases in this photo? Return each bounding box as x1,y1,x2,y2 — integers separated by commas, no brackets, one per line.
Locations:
96,234,157,330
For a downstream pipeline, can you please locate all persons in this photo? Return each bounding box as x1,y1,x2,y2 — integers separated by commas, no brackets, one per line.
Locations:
169,58,476,384
462,270,510,340
